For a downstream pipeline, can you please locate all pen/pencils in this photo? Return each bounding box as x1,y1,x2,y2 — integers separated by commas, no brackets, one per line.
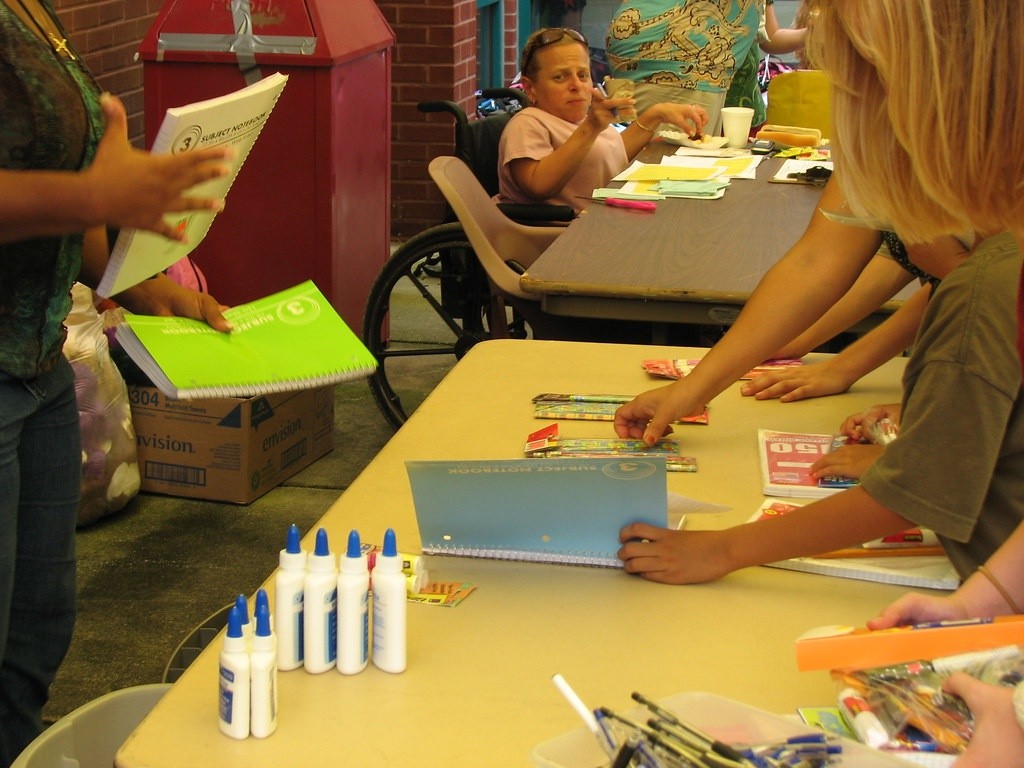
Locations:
673,359,801,378
601,704,707,768
596,82,620,120
632,690,757,768
646,720,746,768
532,437,697,472
733,732,846,768
911,615,993,629
593,709,621,753
805,545,947,560
568,395,636,404
551,672,614,761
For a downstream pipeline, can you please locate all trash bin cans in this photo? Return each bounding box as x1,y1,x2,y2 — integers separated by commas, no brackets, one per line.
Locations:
133,0,394,351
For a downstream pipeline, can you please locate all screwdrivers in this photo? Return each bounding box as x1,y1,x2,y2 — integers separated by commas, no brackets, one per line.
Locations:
573,195,657,210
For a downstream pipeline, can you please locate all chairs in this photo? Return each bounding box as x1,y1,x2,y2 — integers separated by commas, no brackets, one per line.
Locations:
424,155,568,312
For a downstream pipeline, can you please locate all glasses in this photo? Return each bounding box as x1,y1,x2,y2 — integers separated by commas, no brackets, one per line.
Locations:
523,28,589,75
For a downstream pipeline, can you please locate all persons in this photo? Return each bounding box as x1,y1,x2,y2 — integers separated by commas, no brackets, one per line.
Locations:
0,0,233,768
614,0,1024,768
498,27,709,214
605,0,809,136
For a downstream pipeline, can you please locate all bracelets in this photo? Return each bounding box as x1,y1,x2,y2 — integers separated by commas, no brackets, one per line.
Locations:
635,118,657,133
977,566,1021,615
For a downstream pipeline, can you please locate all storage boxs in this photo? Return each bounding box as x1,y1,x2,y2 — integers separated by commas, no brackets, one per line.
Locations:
134,381,341,509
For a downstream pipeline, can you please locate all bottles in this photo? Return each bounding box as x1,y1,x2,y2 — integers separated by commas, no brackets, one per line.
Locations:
219,589,278,738
336,529,370,674
274,522,307,673
303,529,337,673
371,528,406,674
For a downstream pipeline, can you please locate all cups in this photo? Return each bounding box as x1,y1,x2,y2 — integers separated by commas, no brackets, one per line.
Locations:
720,106,754,148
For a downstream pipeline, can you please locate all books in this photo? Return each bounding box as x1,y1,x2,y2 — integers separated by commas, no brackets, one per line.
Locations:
531,394,710,425
743,497,961,593
114,278,378,399
767,157,835,187
96,71,290,299
757,429,913,499
403,455,690,568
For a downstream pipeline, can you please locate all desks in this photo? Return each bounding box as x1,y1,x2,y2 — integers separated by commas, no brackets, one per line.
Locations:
517,129,940,344
104,335,955,768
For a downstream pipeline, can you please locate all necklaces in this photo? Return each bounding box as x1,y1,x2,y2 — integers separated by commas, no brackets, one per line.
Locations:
10,0,76,61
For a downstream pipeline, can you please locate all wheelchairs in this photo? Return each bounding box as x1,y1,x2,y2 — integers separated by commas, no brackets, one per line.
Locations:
361,89,596,432
